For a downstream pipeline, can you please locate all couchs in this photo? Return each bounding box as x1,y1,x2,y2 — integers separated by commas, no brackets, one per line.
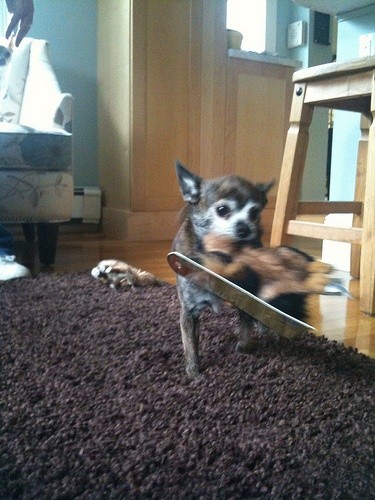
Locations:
0,37,73,264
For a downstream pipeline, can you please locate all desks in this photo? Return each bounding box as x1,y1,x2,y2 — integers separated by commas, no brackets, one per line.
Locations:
271,55,375,316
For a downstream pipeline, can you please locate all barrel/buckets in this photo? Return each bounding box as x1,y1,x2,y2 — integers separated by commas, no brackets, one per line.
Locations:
226,29,244,50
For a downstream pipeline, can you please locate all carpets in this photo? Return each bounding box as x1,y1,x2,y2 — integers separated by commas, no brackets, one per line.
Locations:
0,268,375,500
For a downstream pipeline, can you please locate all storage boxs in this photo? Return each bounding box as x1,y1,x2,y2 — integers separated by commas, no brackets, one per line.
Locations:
73,185,102,224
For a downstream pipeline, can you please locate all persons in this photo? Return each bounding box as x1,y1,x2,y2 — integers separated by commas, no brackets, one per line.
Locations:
0,0,34,48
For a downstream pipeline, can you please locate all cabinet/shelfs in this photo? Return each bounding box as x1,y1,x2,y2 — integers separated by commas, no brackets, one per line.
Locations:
97,0,303,241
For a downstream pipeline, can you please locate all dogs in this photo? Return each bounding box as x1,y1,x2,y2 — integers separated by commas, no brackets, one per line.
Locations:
170,159,355,378
